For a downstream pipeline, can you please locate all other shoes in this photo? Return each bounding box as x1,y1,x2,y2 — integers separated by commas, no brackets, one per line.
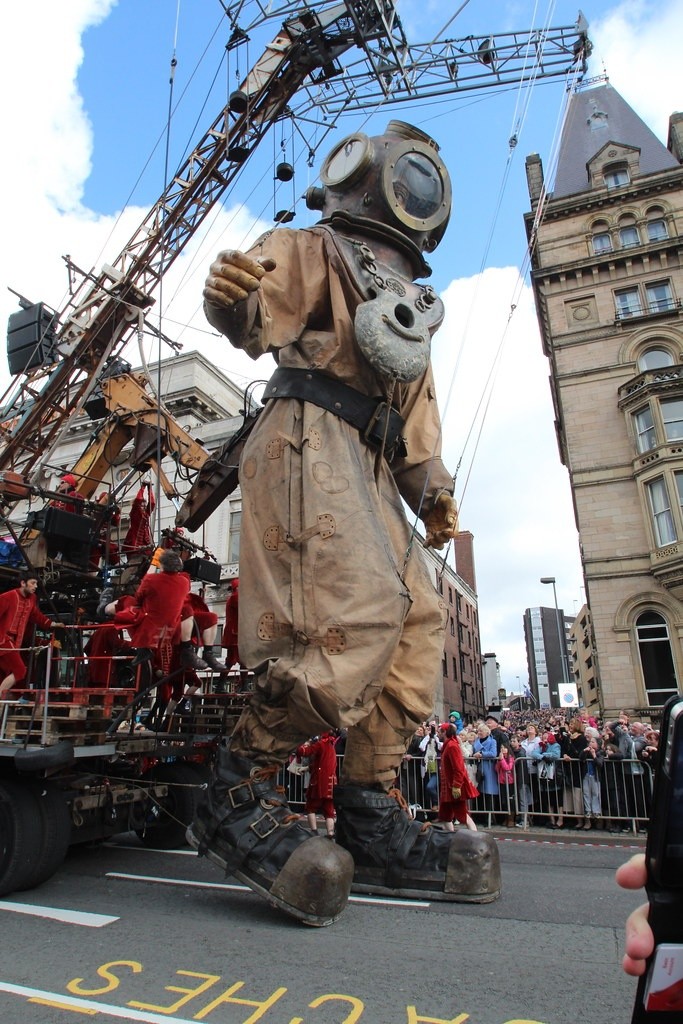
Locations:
324,834,337,840
585,811,601,819
175,704,185,714
142,715,171,732
311,830,318,836
215,687,228,694
204,649,226,671
184,645,207,670
594,822,648,837
240,687,252,693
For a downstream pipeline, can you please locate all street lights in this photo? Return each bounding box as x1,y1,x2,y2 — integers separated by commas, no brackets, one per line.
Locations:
516,676,522,711
540,577,569,683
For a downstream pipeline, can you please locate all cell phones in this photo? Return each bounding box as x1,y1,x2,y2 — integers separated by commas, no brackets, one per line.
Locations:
645,693,683,896
560,726,566,734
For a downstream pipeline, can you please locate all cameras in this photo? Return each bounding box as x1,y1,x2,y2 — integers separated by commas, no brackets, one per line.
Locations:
555,716,562,720
621,724,626,729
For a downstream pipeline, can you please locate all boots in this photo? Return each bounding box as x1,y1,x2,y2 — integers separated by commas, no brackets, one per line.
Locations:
503,811,515,828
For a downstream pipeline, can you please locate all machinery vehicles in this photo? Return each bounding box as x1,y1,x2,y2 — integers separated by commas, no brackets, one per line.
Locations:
0,0,593,893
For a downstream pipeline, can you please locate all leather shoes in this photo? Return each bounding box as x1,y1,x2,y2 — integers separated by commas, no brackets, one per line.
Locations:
548,822,565,829
573,821,592,831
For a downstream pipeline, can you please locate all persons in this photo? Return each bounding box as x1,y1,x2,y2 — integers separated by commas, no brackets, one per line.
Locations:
0,573,66,700
403,707,659,834
297,731,338,844
86,491,125,577
202,116,502,923
83,526,227,724
434,723,477,832
45,474,86,559
214,575,251,693
297,726,348,810
120,475,156,582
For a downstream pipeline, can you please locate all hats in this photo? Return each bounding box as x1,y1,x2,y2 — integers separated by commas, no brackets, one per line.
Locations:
448,710,460,719
61,474,75,486
485,715,499,722
229,579,239,589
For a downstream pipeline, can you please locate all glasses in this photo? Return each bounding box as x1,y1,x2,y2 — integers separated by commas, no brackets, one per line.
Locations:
60,480,67,484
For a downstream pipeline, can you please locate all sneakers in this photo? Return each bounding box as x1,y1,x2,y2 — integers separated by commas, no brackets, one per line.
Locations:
97,587,114,617
515,822,530,828
132,648,153,666
